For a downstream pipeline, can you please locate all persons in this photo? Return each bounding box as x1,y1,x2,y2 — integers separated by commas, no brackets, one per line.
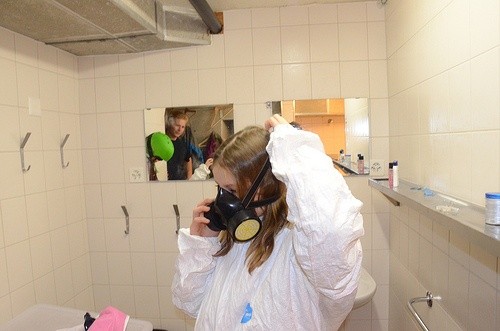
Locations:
164,111,213,181
171,114,365,331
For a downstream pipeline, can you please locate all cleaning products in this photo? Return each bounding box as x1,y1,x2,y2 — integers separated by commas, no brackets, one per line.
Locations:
356,154,364,173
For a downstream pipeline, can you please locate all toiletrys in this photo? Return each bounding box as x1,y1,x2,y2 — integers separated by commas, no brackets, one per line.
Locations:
389,160,399,188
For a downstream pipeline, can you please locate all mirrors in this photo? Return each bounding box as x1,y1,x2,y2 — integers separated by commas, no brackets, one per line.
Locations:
265,98,371,177
142,102,234,181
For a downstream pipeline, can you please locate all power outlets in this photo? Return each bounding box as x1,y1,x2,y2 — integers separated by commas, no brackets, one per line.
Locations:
129,167,145,183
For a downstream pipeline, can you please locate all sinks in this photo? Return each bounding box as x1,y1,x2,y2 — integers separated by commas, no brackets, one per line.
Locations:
337,265,377,331
